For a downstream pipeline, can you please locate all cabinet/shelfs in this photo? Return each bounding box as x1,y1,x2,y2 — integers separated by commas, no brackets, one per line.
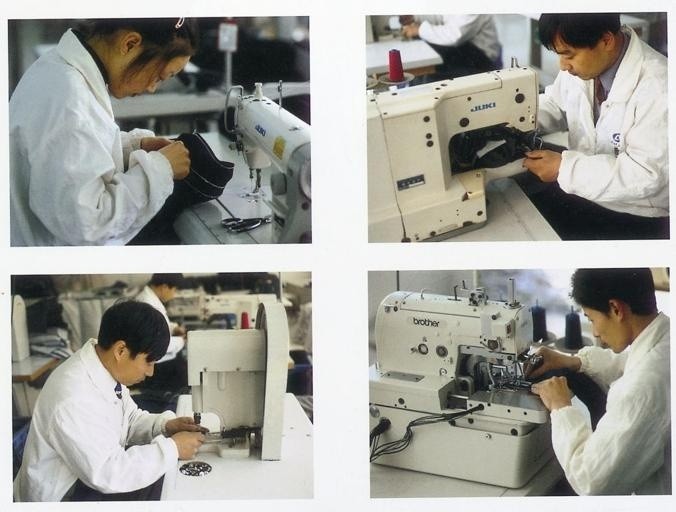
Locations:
495,13,649,91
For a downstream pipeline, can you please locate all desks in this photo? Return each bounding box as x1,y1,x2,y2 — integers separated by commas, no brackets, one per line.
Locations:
151,132,270,244
370,455,565,497
12,356,59,418
438,149,564,242
366,31,444,90
159,393,313,501
31,43,310,133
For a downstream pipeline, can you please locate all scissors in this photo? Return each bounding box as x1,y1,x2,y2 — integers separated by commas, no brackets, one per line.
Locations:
220,214,272,233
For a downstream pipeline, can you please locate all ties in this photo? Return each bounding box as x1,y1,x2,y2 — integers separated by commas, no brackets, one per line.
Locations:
594,76,605,106
116,382,122,399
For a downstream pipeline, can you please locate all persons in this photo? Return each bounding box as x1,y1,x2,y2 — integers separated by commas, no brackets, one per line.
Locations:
13,295,206,501
521,268,672,496
401,15,499,85
522,12,667,239
135,273,190,390
9,16,199,246
